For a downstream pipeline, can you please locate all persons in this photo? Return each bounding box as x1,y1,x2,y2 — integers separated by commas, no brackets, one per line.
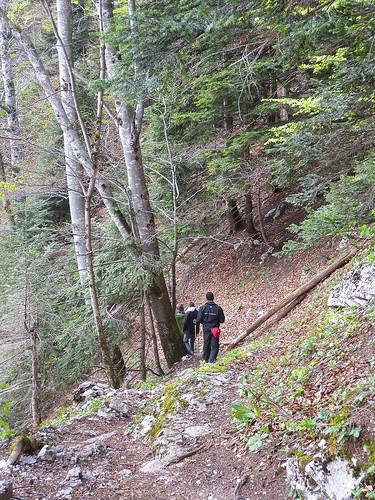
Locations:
196,292,225,363
183,302,200,355
175,303,185,336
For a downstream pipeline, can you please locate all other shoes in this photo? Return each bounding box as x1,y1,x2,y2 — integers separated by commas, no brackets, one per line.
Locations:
190,351,194,355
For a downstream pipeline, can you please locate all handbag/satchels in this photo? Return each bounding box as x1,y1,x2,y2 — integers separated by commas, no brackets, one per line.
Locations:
210,328,221,338
